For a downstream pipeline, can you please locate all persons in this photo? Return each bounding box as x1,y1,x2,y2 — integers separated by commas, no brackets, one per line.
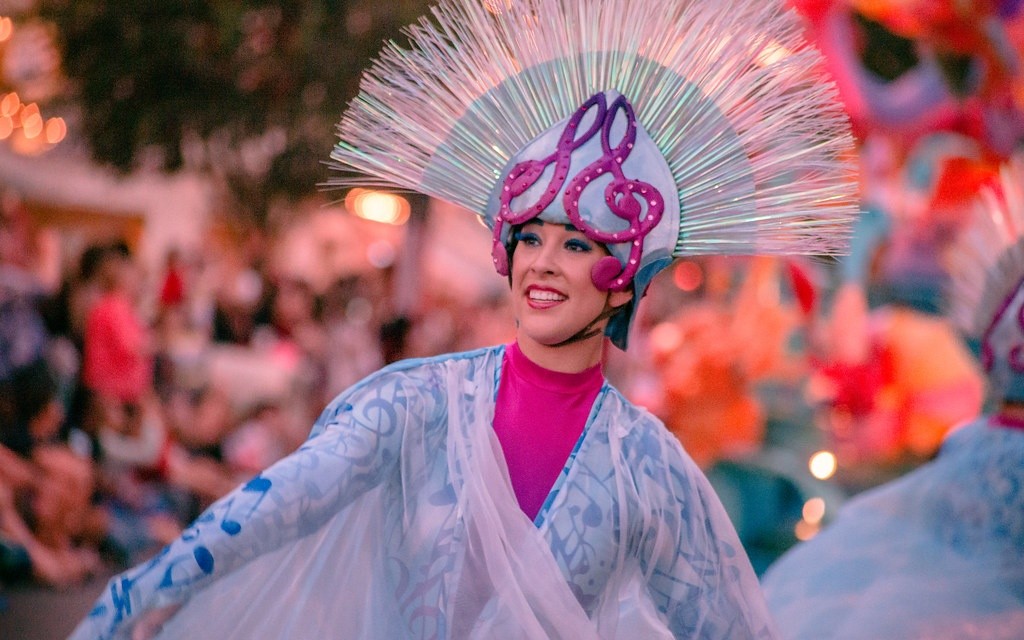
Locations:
67,91,788,640
761,276,1024,640
0,235,510,586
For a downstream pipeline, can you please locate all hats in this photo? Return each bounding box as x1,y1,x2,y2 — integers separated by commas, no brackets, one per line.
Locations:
316,0,861,351
935,152,1024,402
